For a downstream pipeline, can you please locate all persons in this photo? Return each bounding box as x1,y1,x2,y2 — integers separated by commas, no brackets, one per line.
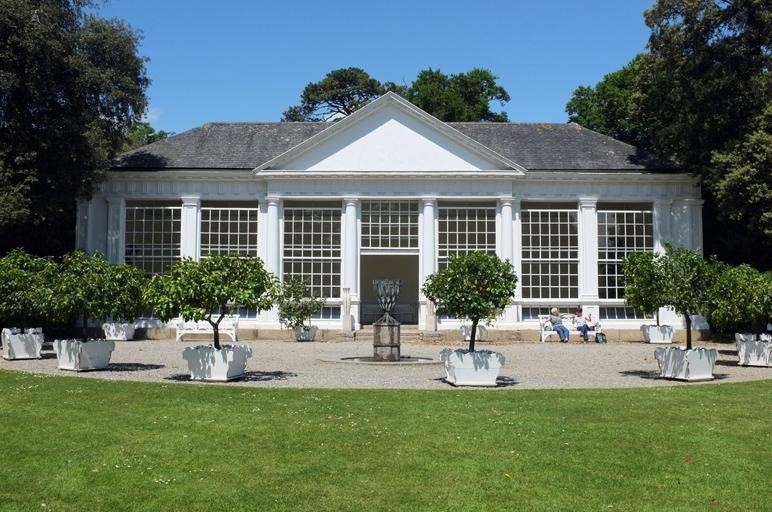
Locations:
572,308,592,343
543,307,569,342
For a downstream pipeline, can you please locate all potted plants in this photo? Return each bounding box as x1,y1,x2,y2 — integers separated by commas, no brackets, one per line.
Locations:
277,271,327,341
421,251,518,386
712,264,772,367
51,248,116,371
623,247,674,344
654,241,718,382
102,263,143,341
143,251,276,382
0,247,51,360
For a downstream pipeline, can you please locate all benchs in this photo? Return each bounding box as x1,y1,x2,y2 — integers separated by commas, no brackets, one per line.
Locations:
538,314,602,342
176,314,240,342
